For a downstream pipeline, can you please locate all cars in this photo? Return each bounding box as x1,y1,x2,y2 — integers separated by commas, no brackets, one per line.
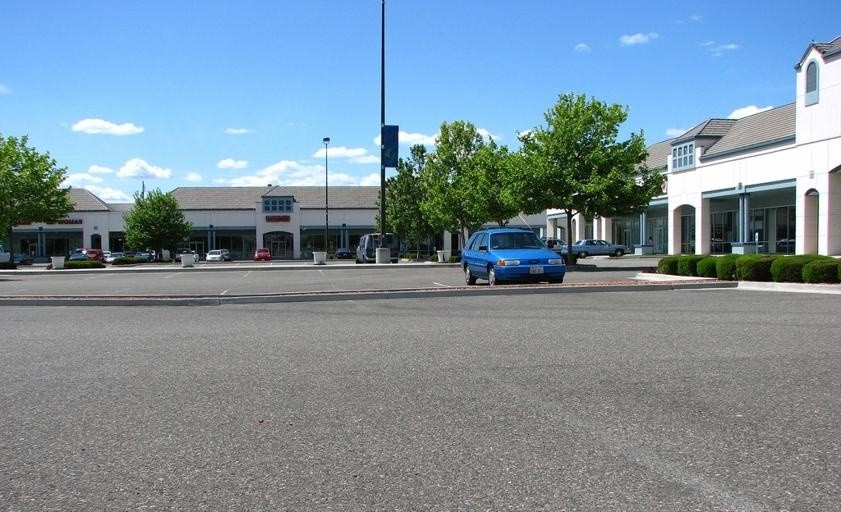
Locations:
13,253,33,265
219,249,230,261
174,247,199,262
64,247,158,266
254,248,271,261
540,236,631,259
461,228,565,285
205,250,223,261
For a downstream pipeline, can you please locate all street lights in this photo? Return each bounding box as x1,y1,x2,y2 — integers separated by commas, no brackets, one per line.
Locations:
321,138,331,251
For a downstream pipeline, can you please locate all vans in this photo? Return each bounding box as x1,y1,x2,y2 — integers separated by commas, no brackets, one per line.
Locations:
355,233,398,264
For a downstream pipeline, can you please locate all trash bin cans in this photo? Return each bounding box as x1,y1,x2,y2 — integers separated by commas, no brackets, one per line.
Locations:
50,256,66,268
312,251,327,265
180,250,194,267
437,250,452,262
